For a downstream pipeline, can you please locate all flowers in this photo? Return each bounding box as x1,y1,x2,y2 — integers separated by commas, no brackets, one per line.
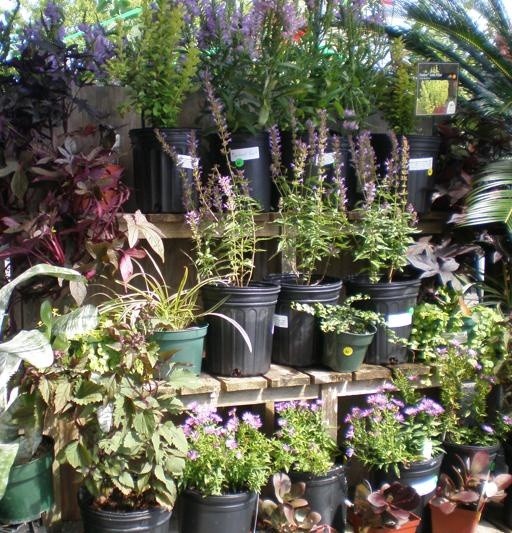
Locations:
345,386,441,434
480,414,510,433
266,0,309,68
342,109,415,217
188,0,262,68
267,97,353,219
155,65,263,224
403,233,496,281
310,1,398,60
176,405,263,460
19,8,114,123
268,398,336,449
440,346,499,392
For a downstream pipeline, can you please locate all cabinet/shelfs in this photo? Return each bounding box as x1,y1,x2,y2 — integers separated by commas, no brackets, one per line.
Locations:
80,211,398,308
44,364,454,455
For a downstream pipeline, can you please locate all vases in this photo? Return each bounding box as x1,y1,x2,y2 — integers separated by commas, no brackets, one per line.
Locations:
177,477,258,530
206,125,267,217
440,433,501,478
274,465,346,525
205,276,273,379
375,129,441,211
373,444,442,514
349,267,422,366
272,270,344,366
272,123,347,207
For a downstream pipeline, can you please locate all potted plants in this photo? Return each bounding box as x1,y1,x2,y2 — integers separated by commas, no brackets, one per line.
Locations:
426,456,507,529
51,382,186,531
264,486,336,533
101,262,210,379
293,297,387,367
352,485,423,533
118,7,197,211
